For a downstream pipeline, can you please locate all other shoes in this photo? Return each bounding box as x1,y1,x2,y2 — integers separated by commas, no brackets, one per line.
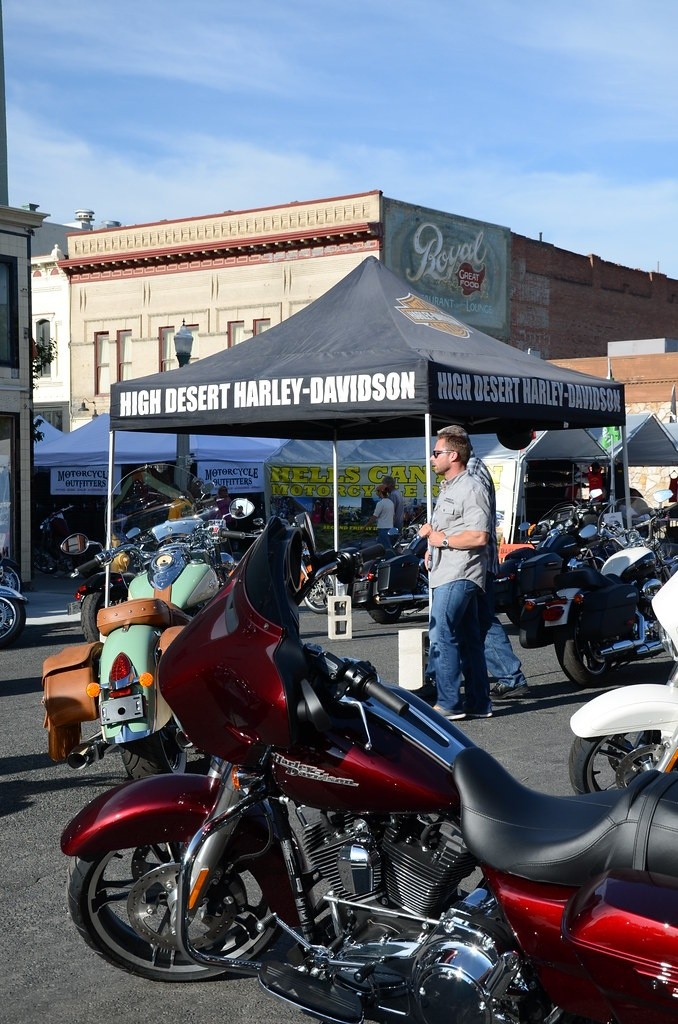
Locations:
460,703,493,718
487,680,532,700
409,682,439,707
430,702,466,721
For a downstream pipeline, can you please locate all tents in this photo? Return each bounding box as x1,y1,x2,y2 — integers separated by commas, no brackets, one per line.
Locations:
102,254,632,609
264,428,613,544
588,412,678,512
33,413,281,495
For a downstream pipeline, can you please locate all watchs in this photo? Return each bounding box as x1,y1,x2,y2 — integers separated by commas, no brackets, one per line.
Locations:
442,535,449,549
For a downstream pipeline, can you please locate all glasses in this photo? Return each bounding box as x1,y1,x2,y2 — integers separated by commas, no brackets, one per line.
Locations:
220,489,227,492
432,450,454,458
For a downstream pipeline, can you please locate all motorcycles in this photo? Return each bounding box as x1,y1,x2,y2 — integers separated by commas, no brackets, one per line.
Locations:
0,556,28,646
568,571,678,802
523,484,678,686
305,499,628,629
59,512,678,1024
31,503,104,577
42,463,255,779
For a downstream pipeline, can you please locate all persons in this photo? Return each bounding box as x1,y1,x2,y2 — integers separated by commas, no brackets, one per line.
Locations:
404,510,410,523
363,476,404,548
587,462,604,502
312,500,322,524
413,502,426,524
215,486,233,556
235,505,245,517
563,462,582,498
324,499,334,523
418,425,531,721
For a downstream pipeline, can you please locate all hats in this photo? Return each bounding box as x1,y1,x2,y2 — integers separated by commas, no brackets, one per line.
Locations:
376,485,389,492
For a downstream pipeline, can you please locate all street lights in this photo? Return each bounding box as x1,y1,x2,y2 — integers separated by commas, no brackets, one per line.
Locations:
173,318,194,490
